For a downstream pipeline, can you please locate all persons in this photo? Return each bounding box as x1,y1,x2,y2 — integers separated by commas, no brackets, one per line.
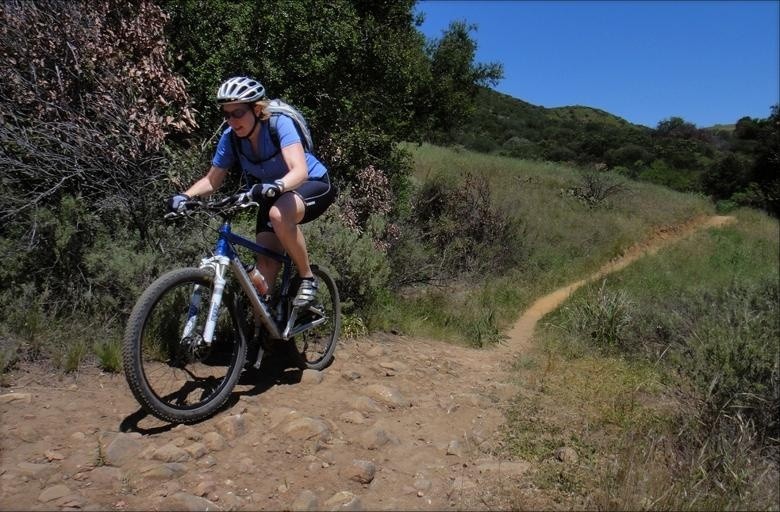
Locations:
163,76,334,368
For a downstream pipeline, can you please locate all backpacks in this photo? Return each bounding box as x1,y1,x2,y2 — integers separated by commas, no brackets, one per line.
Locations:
232,95,313,168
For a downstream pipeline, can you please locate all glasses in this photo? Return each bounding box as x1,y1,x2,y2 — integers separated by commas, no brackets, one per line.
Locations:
219,104,252,122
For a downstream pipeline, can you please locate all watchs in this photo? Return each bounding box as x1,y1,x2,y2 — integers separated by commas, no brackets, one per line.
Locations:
274,178,287,194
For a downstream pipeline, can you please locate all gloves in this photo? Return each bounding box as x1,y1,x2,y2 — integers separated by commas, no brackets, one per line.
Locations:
161,193,193,216
247,178,281,199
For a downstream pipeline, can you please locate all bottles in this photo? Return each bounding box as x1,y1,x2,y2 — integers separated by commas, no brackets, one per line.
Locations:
244,263,269,296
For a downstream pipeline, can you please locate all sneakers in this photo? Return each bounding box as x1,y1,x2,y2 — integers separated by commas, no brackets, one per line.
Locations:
294,273,319,310
233,339,269,367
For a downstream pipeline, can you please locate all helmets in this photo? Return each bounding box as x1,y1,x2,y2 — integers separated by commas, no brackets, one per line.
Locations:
214,75,267,107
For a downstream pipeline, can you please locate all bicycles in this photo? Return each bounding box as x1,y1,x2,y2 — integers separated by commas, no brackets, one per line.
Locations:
123,187,344,424
